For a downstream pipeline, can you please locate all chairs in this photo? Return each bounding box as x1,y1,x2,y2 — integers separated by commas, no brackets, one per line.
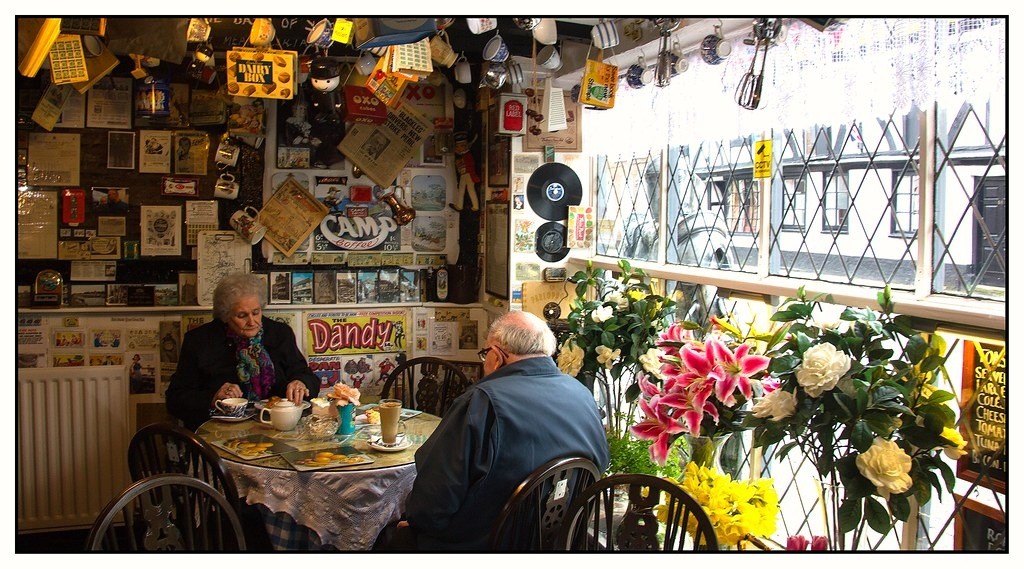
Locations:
84,356,720,553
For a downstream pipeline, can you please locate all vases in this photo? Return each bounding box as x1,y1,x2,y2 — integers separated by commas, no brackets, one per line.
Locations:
676,431,733,483
334,402,356,435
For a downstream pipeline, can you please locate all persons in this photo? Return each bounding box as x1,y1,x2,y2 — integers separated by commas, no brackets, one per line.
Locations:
100,190,127,214
377,310,610,554
303,56,347,167
176,137,195,173
447,131,480,213
166,268,321,434
188,43,214,85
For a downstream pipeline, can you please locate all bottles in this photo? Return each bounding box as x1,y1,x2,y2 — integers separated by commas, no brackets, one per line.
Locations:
431,255,450,302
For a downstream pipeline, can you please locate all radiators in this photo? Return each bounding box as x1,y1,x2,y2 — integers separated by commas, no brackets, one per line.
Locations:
17,364,133,535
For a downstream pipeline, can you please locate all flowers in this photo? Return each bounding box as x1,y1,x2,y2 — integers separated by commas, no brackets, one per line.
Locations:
557,258,968,554
326,382,362,407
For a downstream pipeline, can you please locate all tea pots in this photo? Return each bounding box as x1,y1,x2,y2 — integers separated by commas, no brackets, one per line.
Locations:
260,400,303,431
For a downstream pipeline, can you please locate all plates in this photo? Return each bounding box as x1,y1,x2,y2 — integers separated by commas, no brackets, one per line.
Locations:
211,411,255,422
254,398,312,411
366,436,412,451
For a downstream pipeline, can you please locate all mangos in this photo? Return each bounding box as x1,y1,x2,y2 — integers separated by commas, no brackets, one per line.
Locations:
224,441,273,451
313,452,347,462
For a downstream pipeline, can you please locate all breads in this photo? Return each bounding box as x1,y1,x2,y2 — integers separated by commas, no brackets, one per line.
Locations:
227,52,292,97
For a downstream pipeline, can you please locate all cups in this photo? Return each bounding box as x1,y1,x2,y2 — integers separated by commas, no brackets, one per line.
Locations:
214,173,240,199
215,398,249,418
215,143,240,170
379,399,407,447
185,16,732,95
229,205,269,245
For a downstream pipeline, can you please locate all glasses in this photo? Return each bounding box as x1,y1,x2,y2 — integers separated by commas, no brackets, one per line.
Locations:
477,346,509,362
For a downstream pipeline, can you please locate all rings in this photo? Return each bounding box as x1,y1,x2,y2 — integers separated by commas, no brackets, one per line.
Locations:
225,392,228,394
294,389,299,392
228,387,231,391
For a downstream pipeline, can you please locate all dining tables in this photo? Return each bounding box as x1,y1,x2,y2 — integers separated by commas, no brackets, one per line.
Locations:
182,401,443,552
